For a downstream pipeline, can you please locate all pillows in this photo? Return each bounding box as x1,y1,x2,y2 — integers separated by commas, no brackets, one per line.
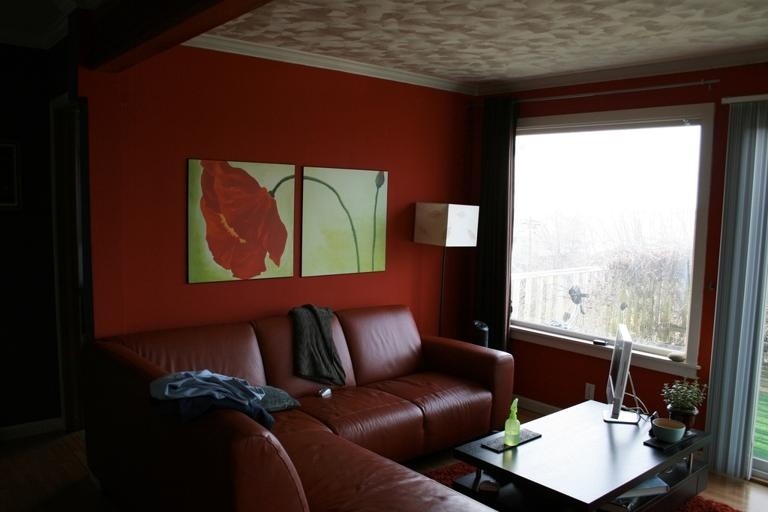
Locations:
257,383,302,412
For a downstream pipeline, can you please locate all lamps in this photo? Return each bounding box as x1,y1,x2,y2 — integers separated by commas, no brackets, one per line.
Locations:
414,201,480,336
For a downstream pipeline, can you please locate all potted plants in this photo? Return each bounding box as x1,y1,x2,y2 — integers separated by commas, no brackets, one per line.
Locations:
660,375,710,432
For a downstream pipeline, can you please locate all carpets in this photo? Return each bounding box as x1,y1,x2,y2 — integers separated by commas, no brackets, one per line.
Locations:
420,460,743,511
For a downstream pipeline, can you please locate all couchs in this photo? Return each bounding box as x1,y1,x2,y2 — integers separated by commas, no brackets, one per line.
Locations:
95,304,515,511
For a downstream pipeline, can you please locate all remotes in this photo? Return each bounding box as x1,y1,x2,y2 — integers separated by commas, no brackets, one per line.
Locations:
593,339,606,345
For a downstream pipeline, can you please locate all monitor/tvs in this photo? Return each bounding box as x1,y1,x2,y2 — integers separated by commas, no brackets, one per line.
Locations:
602,323,641,424
